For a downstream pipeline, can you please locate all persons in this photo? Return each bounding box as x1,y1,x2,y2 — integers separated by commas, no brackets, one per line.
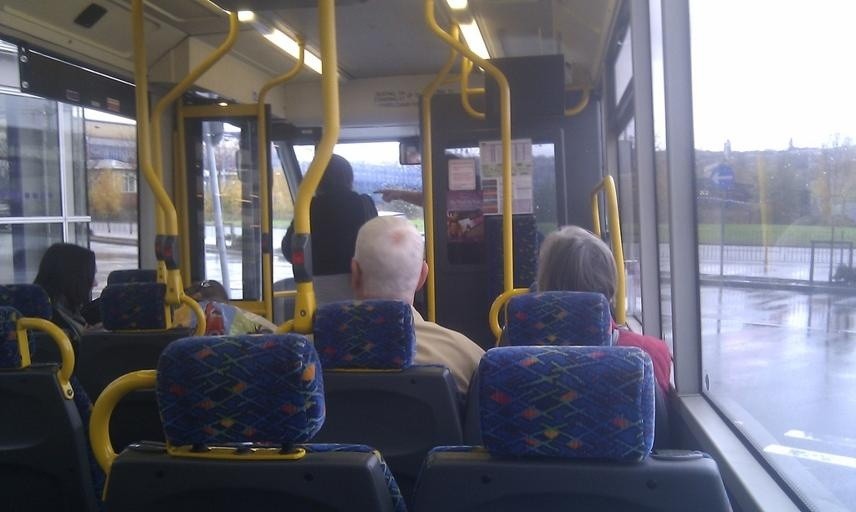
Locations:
281,154,377,307
496,226,671,402
351,216,486,399
373,187,424,206
33,242,106,335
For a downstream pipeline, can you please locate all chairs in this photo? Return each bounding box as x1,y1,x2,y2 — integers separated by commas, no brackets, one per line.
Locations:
304,191,367,273
1,269,732,511
481,207,539,265
